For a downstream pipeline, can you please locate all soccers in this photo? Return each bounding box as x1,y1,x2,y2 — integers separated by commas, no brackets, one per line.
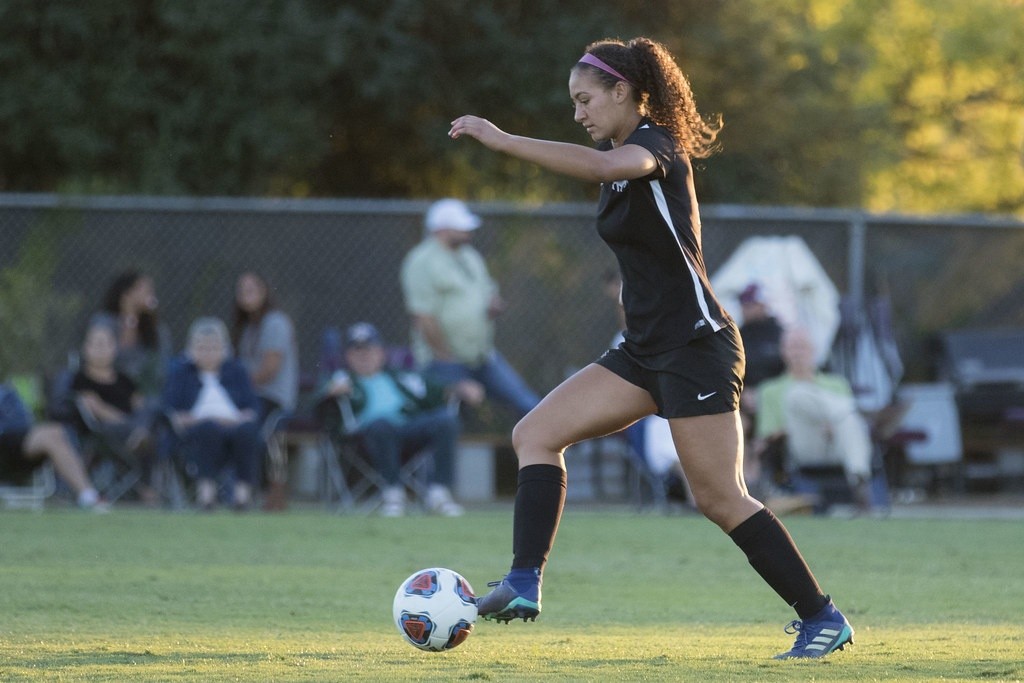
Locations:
389,564,480,652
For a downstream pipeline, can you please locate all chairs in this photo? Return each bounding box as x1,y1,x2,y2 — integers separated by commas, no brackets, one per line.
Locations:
757,358,889,513
630,421,760,519
157,349,265,510
318,327,471,523
41,348,170,510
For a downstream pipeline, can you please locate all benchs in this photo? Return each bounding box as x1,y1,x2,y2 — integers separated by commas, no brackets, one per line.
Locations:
284,429,512,506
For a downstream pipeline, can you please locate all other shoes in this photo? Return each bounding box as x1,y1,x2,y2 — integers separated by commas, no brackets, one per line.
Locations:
417,483,463,517
382,488,405,520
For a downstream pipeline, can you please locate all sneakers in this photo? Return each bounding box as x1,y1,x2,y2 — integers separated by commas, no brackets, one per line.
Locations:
773,594,854,660
476,568,543,624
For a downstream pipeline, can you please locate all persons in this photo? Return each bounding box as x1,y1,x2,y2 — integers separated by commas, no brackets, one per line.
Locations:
0,200,547,513
614,270,962,515
449,38,854,661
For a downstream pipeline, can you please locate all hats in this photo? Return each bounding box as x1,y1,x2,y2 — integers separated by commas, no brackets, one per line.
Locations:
426,198,482,232
340,321,379,351
